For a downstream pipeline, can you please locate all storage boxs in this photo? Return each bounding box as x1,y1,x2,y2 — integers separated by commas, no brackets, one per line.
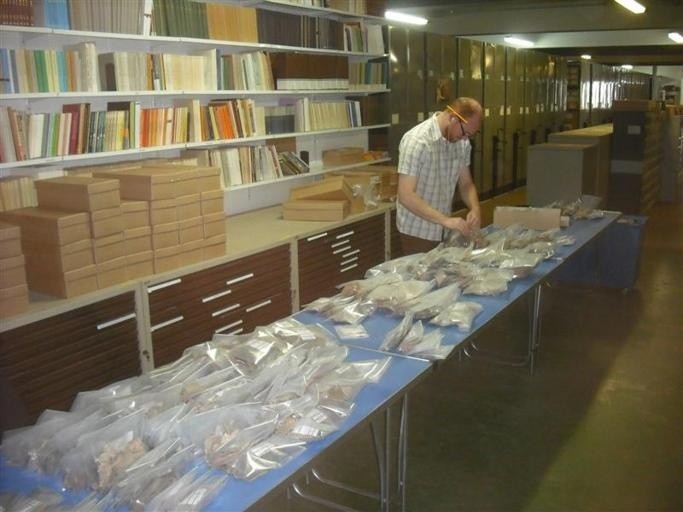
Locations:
283,146,398,221
0,162,226,322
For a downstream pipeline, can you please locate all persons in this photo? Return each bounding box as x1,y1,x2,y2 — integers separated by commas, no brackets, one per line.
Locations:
395,96,483,255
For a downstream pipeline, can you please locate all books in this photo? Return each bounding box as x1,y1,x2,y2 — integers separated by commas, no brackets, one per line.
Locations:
180,144,283,184
256,1,393,177
282,165,393,218
0,174,37,210
3,1,259,165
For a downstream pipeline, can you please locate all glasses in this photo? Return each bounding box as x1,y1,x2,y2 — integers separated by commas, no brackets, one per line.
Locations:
457,116,470,139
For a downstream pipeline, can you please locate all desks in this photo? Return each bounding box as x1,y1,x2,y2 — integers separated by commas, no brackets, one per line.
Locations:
0,328,434,512
